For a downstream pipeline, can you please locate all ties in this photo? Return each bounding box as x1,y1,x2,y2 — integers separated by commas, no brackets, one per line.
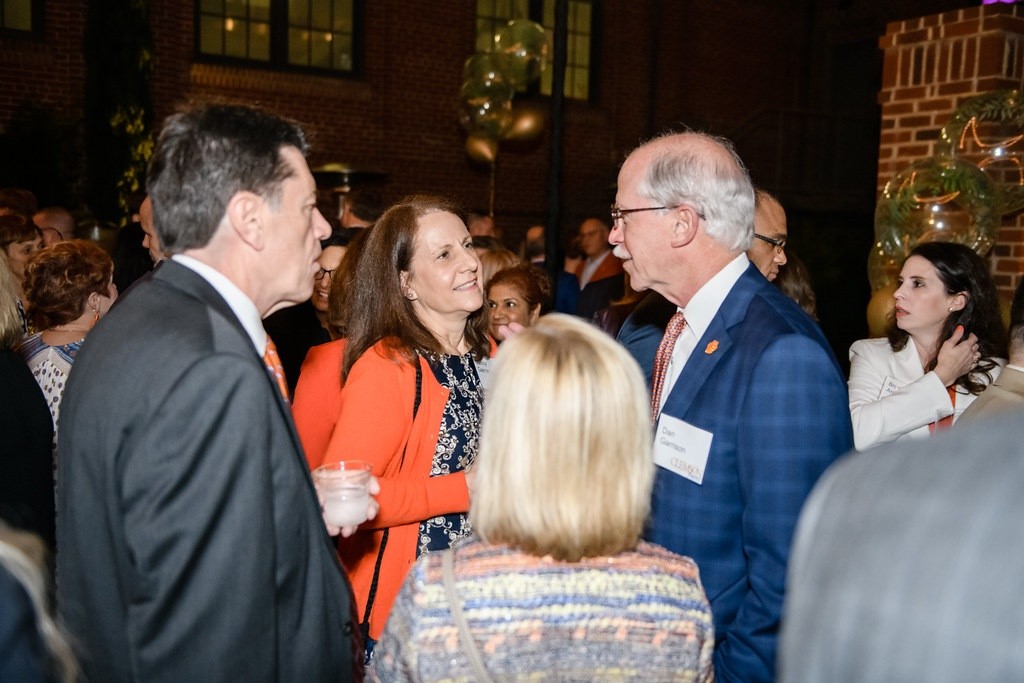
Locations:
649,311,688,425
263,333,289,403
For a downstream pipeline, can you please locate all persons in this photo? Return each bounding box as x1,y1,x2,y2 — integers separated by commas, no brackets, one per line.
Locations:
0,103,1023,683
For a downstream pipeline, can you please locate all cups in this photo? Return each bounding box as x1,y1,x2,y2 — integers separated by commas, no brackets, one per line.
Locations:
312,459,372,527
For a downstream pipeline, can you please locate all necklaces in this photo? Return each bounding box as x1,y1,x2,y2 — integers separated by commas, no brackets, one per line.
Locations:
47,327,85,333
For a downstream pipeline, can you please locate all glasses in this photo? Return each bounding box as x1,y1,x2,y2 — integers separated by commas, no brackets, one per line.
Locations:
754,232,786,253
612,205,706,229
314,267,337,280
580,227,606,241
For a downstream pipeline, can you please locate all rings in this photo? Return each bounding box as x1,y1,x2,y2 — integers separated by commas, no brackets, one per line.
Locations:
975,354,979,363
